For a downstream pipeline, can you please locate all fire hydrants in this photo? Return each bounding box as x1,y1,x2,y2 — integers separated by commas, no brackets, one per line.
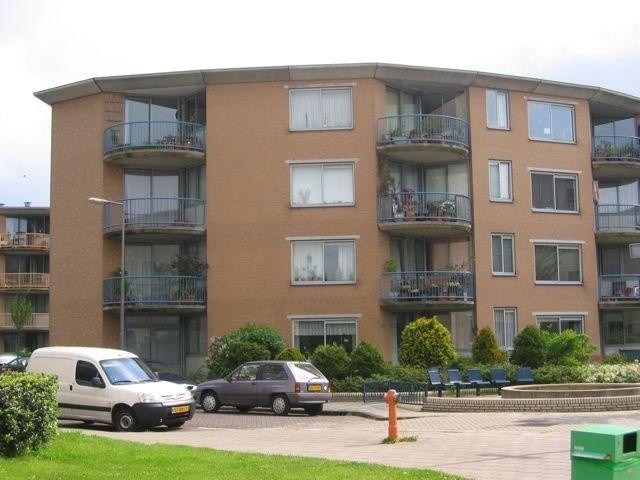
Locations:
383,388,400,440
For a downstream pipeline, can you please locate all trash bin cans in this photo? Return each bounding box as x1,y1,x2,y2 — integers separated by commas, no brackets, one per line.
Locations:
570,424,640,480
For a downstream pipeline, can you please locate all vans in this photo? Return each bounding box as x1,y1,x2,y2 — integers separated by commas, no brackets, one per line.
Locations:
27,345,195,432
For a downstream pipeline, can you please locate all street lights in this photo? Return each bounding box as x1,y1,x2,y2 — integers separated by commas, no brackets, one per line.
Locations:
88,197,125,345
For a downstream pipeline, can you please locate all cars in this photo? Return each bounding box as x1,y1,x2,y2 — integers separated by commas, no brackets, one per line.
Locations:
0,353,27,372
197,360,330,415
143,360,196,399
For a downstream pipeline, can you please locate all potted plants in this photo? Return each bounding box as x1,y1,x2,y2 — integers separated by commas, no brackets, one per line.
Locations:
376,155,468,223
588,134,639,165
375,109,467,149
383,258,471,299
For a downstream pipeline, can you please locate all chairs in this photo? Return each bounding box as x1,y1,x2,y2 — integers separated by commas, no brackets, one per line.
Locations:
424,366,534,398
